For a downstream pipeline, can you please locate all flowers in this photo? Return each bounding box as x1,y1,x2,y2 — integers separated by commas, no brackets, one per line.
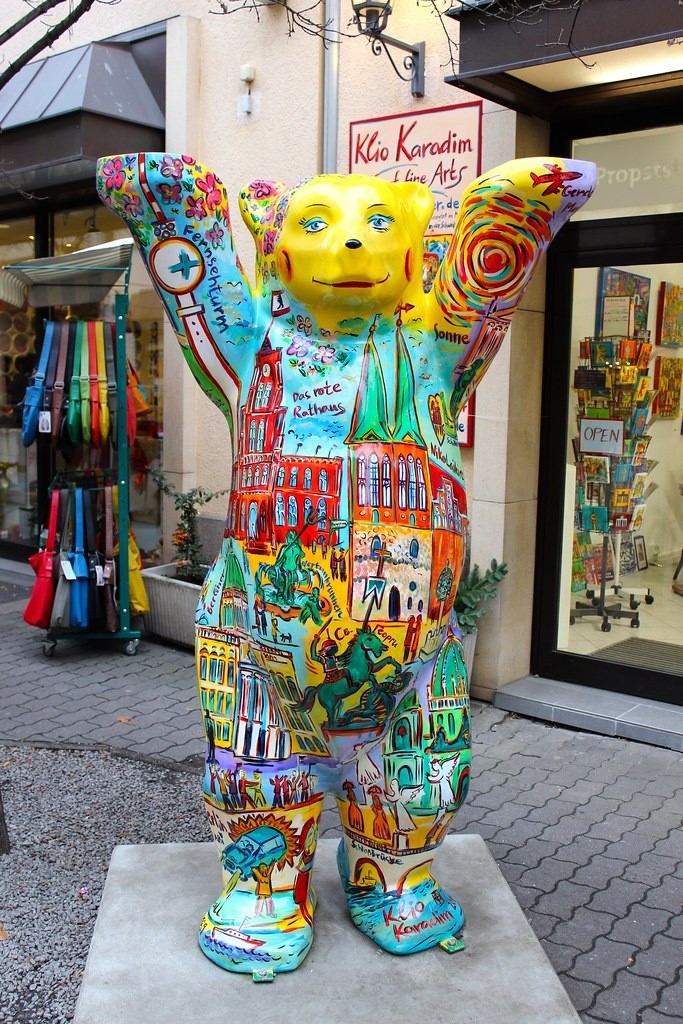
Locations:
145,464,232,584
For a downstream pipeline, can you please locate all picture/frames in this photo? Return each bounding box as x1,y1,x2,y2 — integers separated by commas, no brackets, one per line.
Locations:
634,536,648,571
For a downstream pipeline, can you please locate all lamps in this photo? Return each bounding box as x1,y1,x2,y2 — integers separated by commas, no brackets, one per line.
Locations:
352,0,425,98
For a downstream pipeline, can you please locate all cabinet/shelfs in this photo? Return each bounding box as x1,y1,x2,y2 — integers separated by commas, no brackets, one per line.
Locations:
0,405,51,565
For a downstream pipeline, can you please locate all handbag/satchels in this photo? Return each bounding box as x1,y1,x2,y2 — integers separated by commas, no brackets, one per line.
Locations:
20,322,149,449
23,485,150,633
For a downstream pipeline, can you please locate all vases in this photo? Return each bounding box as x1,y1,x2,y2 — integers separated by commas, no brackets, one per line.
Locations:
138,560,212,647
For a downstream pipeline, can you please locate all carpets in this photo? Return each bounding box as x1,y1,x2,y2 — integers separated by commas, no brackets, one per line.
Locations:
589,637,683,676
